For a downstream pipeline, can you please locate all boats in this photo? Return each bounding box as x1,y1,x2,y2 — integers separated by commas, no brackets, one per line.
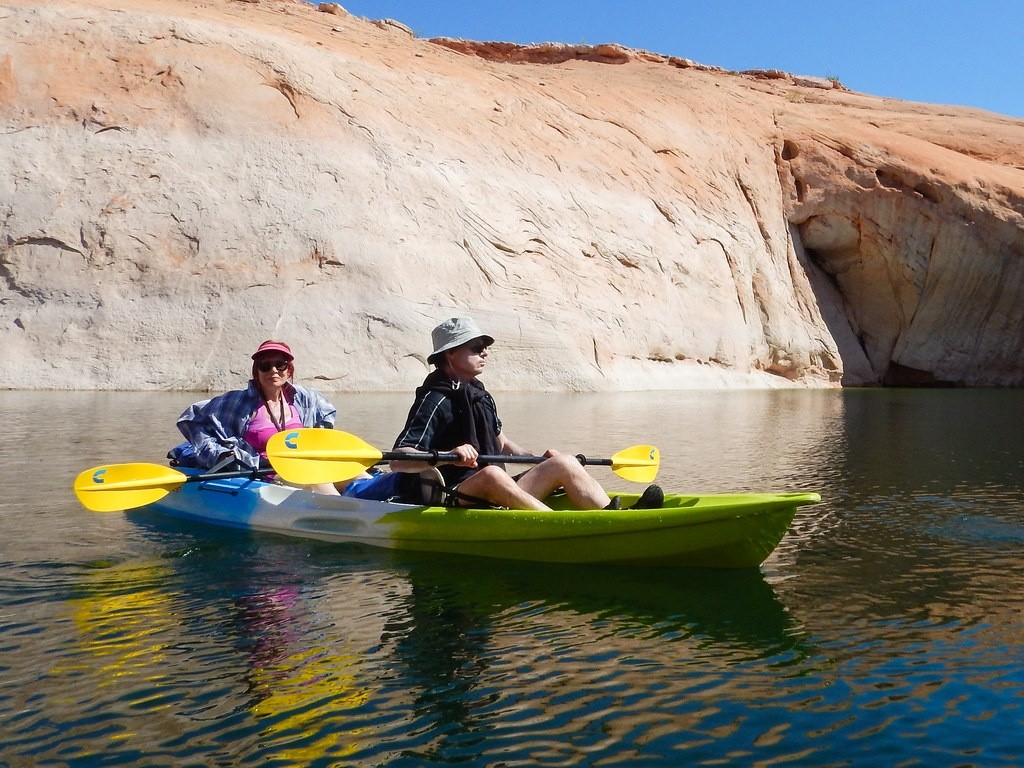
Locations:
147,466,821,570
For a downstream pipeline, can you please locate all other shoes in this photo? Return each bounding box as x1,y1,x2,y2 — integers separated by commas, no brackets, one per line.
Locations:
602,484,664,510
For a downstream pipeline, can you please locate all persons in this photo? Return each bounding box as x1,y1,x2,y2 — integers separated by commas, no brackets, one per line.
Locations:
177,339,373,496
388,316,664,512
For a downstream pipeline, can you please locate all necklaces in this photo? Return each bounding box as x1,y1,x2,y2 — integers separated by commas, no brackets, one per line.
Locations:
263,398,286,432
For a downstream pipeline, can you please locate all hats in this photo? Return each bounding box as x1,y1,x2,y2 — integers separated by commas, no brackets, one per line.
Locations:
427,316,495,364
252,340,295,360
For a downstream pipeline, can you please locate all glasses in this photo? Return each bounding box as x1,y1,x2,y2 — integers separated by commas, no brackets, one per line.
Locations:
468,343,487,353
255,360,288,373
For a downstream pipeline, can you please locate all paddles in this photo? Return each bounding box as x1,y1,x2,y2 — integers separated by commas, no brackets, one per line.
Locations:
262,426,664,486
72,449,391,514
